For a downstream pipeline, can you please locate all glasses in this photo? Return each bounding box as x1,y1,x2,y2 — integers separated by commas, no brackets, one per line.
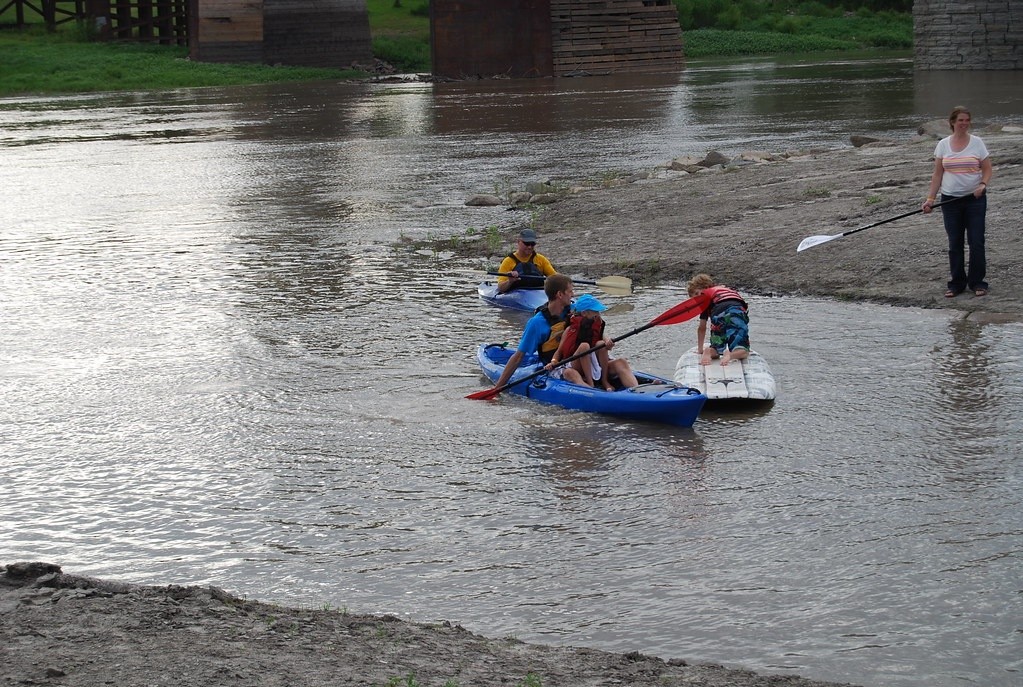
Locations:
522,241,536,247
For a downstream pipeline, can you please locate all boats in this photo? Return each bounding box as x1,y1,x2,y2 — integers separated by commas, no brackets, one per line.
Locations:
476,340,706,427
478,280,578,313
675,342,778,411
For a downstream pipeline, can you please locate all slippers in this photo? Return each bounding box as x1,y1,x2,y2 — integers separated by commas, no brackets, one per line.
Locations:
976,288,987,296
944,290,960,297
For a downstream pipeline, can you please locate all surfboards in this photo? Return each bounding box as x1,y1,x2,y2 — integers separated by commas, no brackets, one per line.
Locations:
673,342,777,401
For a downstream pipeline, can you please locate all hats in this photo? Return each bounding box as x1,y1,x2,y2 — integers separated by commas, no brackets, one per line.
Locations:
576,294,607,312
518,229,536,242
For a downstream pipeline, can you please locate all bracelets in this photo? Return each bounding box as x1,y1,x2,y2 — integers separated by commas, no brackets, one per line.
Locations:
979,181,986,186
551,358,558,362
927,196,935,201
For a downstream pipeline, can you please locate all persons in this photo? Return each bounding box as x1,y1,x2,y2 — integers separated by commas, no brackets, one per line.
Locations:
497,229,575,297
688,273,750,366
922,106,993,298
494,273,661,390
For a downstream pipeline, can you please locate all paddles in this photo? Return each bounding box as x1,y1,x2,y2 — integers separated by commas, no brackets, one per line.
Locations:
454,269,635,296
465,294,710,401
796,187,987,253
